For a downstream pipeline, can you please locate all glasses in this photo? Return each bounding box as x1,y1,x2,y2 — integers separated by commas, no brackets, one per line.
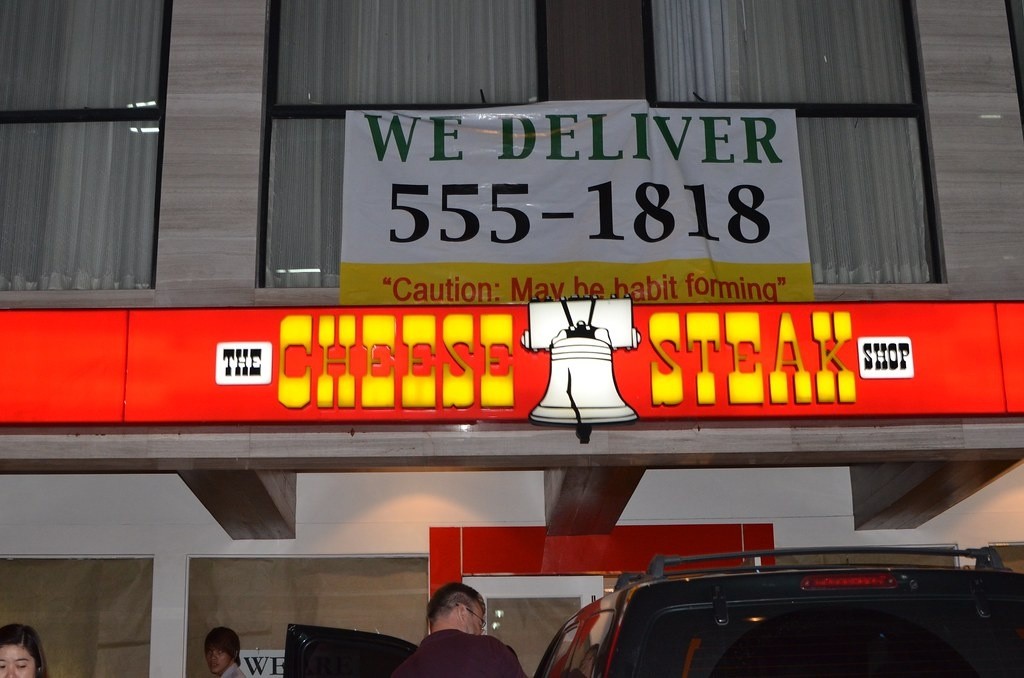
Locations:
455,602,487,628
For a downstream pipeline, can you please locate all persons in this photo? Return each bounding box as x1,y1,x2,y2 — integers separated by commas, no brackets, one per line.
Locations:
390,583,524,678
204,626,246,678
571,644,598,678
0,624,48,678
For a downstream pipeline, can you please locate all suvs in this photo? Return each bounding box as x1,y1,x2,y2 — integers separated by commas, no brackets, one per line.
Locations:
283,542,1024,678
